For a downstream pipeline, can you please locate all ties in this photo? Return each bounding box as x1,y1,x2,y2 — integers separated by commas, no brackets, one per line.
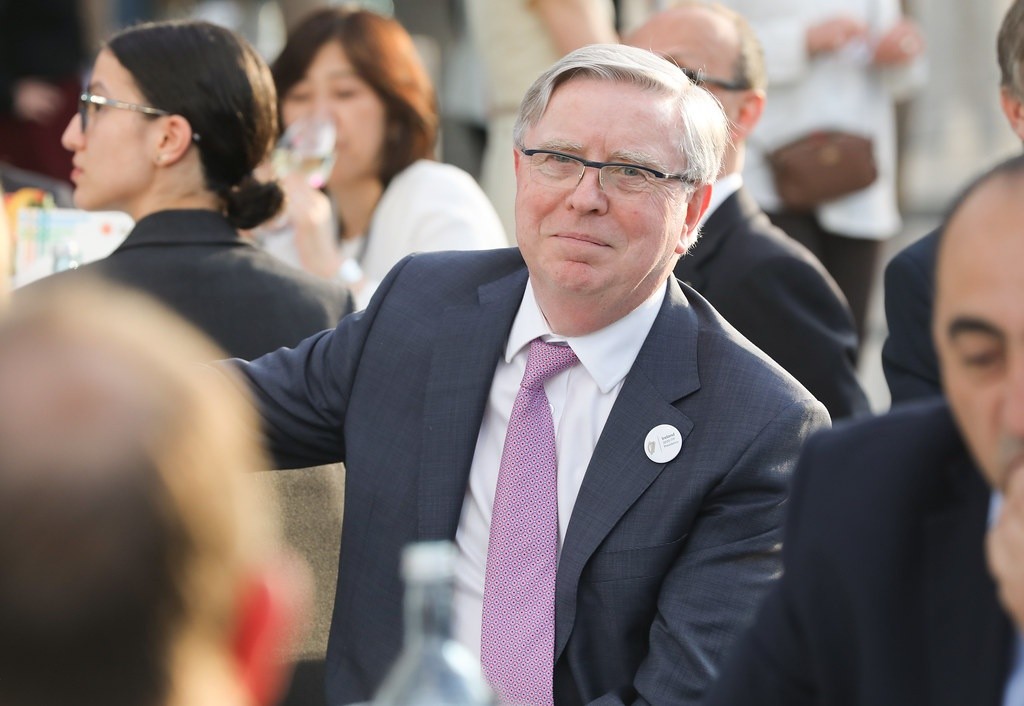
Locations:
478,338,582,706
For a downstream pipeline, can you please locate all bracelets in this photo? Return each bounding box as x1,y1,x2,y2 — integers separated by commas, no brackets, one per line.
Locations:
331,260,362,284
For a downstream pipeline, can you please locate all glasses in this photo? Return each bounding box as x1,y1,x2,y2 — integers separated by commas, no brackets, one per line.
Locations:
514,138,697,201
80,80,201,142
683,67,750,90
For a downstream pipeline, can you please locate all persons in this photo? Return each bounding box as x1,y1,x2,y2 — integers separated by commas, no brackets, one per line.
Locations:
211,44,832,705
696,154,1024,705
627,3,875,425
532,1,931,366
260,9,511,313
879,0,1024,408
0,277,311,706
0,21,355,363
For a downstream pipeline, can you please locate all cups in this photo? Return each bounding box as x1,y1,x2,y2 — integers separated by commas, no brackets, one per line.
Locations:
269,113,341,190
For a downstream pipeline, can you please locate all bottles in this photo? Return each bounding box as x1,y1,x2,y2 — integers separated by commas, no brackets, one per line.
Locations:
366,538,495,705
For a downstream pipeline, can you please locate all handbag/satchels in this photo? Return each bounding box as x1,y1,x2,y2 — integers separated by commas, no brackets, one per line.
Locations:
766,132,874,212
